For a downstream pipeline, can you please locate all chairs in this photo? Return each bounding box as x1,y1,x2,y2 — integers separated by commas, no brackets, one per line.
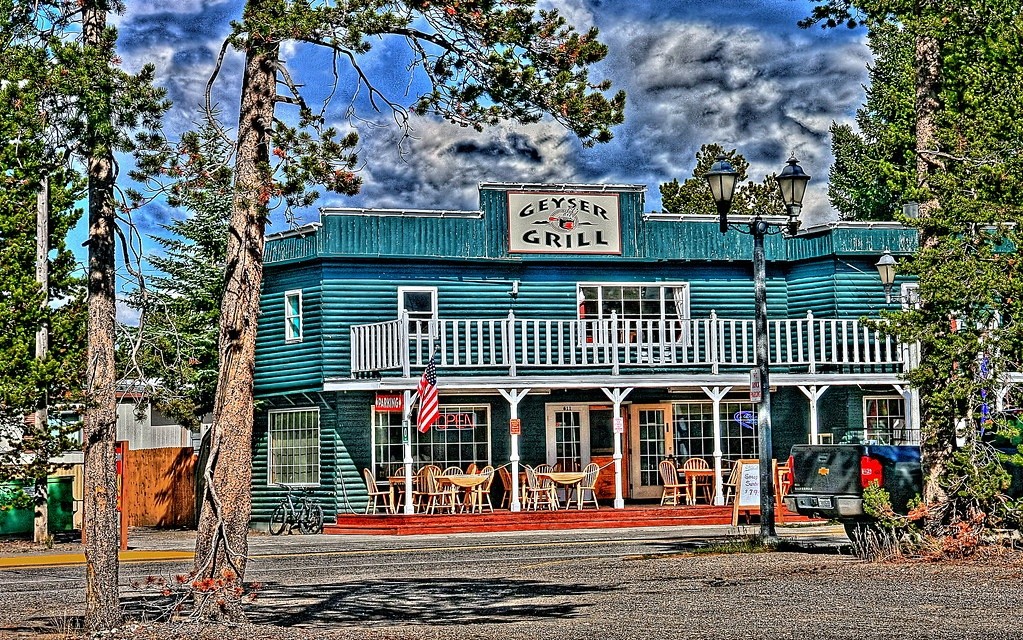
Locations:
363,459,602,519
658,458,791,507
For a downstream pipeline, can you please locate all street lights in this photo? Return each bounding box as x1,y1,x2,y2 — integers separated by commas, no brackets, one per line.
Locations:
703,151,815,544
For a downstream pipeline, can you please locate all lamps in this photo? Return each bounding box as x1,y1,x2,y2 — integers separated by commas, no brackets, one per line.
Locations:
438,389,551,396
667,386,777,394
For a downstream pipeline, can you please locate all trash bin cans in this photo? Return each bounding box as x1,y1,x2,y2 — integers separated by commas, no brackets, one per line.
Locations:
0,475,75,540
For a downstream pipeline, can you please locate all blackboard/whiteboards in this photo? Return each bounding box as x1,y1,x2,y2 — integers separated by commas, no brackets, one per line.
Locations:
733,459,776,511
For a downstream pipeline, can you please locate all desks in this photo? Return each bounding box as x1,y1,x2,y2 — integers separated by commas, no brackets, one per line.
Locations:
386,472,490,515
508,470,585,513
773,467,792,505
675,466,733,506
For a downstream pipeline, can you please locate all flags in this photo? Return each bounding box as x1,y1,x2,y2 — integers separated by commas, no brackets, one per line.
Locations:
417,354,440,433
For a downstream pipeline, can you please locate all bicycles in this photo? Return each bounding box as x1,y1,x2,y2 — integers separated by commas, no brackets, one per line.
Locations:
268,481,325,537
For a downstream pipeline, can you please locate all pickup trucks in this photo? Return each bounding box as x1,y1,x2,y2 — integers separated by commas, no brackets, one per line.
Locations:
779,408,1023,549
